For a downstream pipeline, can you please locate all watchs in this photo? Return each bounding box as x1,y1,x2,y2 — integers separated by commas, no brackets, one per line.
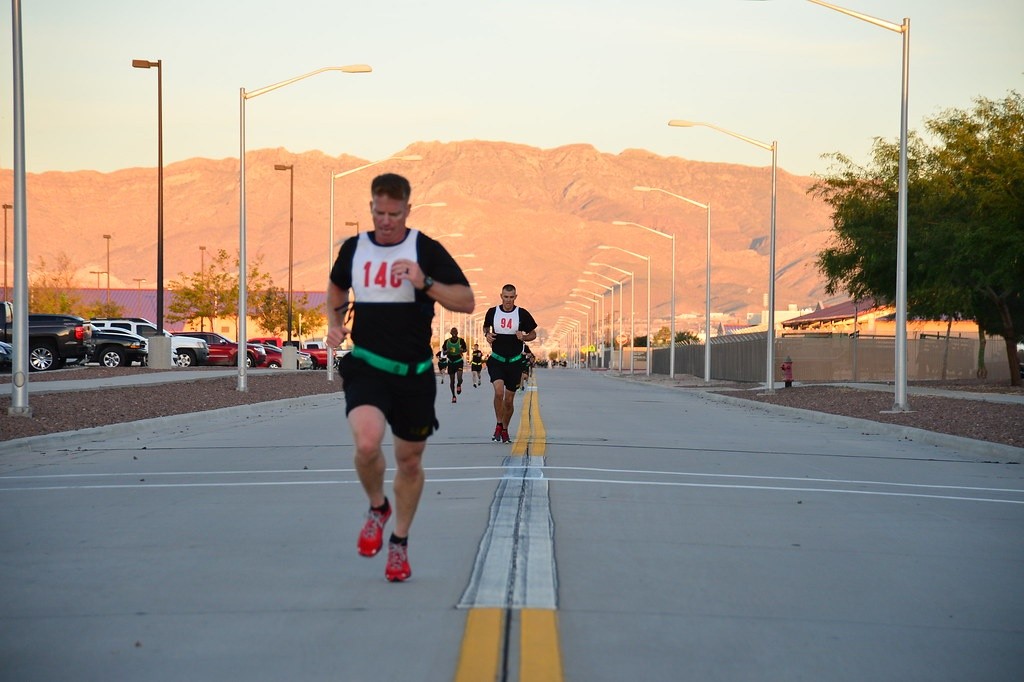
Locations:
484,331,488,337
421,276,434,293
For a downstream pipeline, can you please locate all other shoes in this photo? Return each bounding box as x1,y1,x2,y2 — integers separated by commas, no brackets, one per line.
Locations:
452,397,456,403
457,384,461,394
478,378,481,386
473,384,476,388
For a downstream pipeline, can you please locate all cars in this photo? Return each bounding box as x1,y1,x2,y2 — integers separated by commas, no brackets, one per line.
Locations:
97,325,178,369
27,313,148,368
169,330,352,370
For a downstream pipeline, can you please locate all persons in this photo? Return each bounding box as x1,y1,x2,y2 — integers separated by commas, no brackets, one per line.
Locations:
436,351,448,384
519,341,536,391
326,173,475,584
483,284,538,445
469,344,483,388
443,327,467,403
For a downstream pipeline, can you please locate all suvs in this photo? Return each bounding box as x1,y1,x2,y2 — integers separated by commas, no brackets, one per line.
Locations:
86,316,210,368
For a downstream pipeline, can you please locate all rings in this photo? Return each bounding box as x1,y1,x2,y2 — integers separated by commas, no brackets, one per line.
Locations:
406,268,408,274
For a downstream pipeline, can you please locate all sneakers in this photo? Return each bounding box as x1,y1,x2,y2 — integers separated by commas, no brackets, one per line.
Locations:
385,536,410,581
492,424,502,441
357,497,391,557
501,427,510,444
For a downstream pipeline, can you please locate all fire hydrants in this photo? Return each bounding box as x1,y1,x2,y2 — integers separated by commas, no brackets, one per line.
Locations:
780,356,795,388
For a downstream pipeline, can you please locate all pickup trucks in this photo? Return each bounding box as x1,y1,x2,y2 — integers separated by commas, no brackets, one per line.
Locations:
0,303,93,372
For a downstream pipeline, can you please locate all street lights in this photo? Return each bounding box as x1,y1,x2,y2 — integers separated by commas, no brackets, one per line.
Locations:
90,271,108,289
132,58,163,335
274,165,294,347
199,246,207,332
327,154,424,381
2,203,12,301
610,220,676,378
345,221,360,236
539,243,652,379
633,185,711,382
133,278,146,317
667,119,777,392
103,234,111,304
235,64,371,391
